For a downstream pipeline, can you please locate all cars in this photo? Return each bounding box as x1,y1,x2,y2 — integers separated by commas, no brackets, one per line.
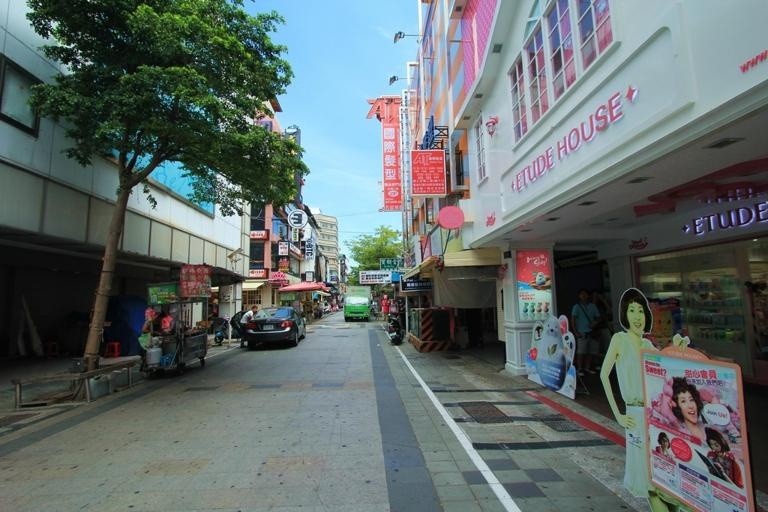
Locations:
229,306,308,347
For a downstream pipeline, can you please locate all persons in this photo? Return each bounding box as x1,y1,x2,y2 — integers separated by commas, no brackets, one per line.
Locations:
597,287,659,501
141,310,166,336
238,305,258,348
569,288,602,378
670,376,708,444
389,299,400,320
655,431,669,458
703,426,743,488
381,294,391,322
313,302,321,318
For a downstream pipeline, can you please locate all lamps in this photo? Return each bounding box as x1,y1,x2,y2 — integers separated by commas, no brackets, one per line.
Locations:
394,31,428,44
389,76,415,86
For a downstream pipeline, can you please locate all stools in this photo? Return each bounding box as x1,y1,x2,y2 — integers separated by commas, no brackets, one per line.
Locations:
104,342,121,357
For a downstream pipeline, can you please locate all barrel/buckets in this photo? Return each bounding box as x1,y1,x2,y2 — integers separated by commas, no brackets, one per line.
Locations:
114,363,141,386
84,353,100,370
88,375,111,397
72,357,87,373
146,348,162,367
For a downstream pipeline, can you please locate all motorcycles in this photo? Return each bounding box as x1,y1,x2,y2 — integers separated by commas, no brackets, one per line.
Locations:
213,314,229,345
386,312,404,344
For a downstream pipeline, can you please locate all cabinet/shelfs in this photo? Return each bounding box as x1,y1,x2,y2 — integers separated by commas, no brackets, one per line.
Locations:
635,243,756,380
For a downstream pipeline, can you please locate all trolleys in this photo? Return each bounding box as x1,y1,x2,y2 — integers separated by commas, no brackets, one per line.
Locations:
145,280,209,377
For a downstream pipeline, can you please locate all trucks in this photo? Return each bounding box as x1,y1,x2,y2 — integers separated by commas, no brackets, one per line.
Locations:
342,285,372,322
